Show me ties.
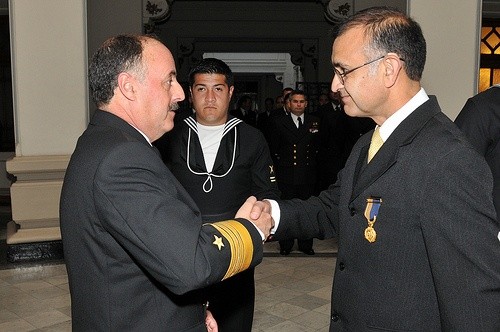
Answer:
[336,105,339,112]
[367,126,382,164]
[297,116,303,129]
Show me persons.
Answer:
[228,87,348,255]
[249,6,500,332]
[161,58,283,332]
[58,33,276,332]
[454,83,500,216]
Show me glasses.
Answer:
[333,55,407,85]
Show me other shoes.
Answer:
[280,249,290,255]
[305,249,314,255]
